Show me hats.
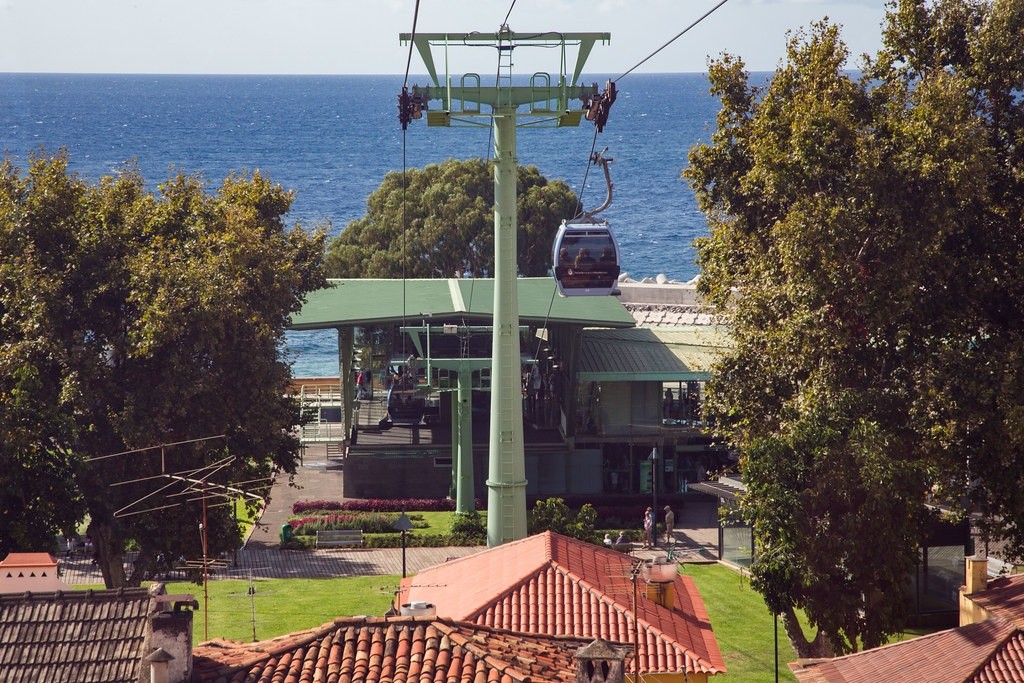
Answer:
[664,506,670,509]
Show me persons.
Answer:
[555,247,572,273]
[599,446,742,497]
[603,532,613,545]
[380,354,562,433]
[663,391,671,423]
[355,370,369,402]
[645,506,657,543]
[616,531,630,544]
[641,511,653,550]
[64,536,192,579]
[665,388,676,420]
[600,246,619,273]
[663,505,679,545]
[574,246,598,271]
[575,248,587,268]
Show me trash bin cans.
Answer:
[282,523,293,545]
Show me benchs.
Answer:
[316,529,363,551]
[986,556,1018,578]
[610,543,634,557]
